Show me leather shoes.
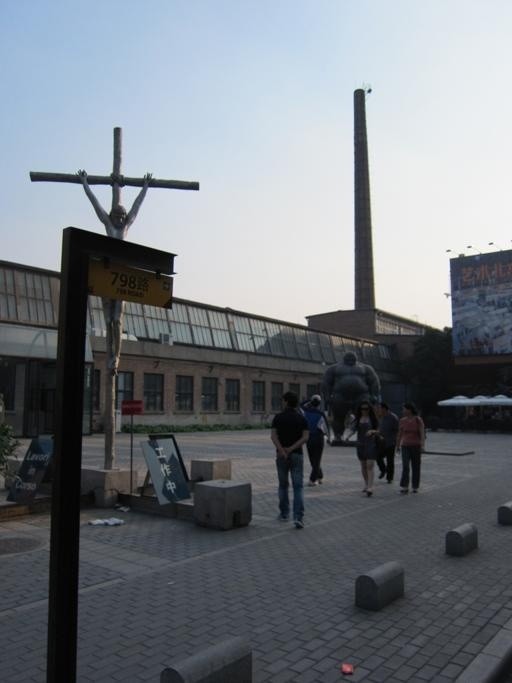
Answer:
[379,467,386,479]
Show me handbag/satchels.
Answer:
[374,432,386,455]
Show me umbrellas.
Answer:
[437,394,512,419]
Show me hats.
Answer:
[311,394,321,404]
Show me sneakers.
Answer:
[293,519,304,528]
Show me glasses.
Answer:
[360,406,368,411]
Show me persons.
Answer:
[394,399,426,494]
[319,350,384,441]
[299,394,330,486]
[344,398,377,497]
[74,166,159,375]
[374,401,399,485]
[270,390,311,529]
